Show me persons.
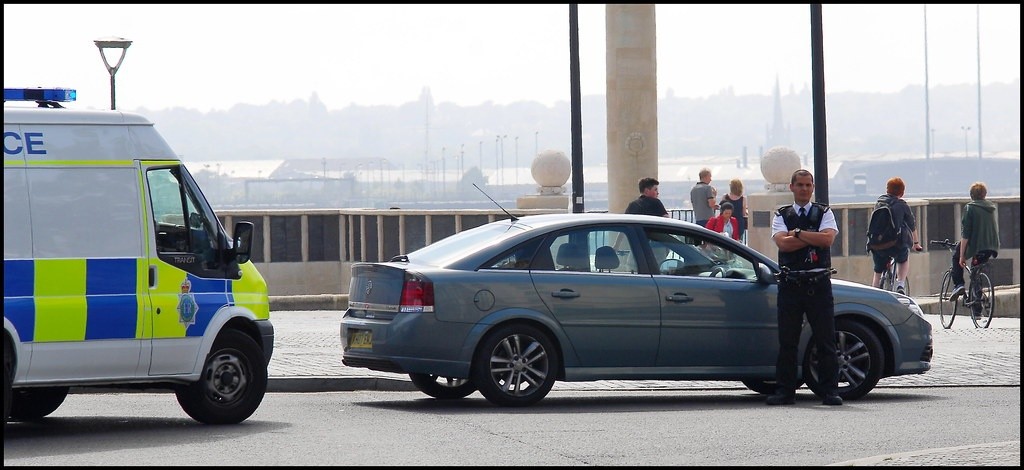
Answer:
[706,200,740,243]
[949,182,1000,320]
[690,168,720,228]
[719,177,747,239]
[767,169,845,406]
[866,178,922,295]
[611,176,671,265]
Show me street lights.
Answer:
[92,35,134,111]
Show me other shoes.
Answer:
[897,285,905,294]
[767,393,794,404]
[823,393,842,405]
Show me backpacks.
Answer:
[866,200,902,252]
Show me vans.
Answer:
[0,87,274,425]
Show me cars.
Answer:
[340,213,933,408]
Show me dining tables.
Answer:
[595,246,620,274]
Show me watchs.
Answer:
[795,228,801,238]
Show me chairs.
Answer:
[556,243,579,272]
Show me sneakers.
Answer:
[971,312,980,319]
[949,285,965,302]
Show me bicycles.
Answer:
[930,239,995,329]
[880,247,923,297]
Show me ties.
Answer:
[799,208,806,221]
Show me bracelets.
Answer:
[914,241,919,244]
